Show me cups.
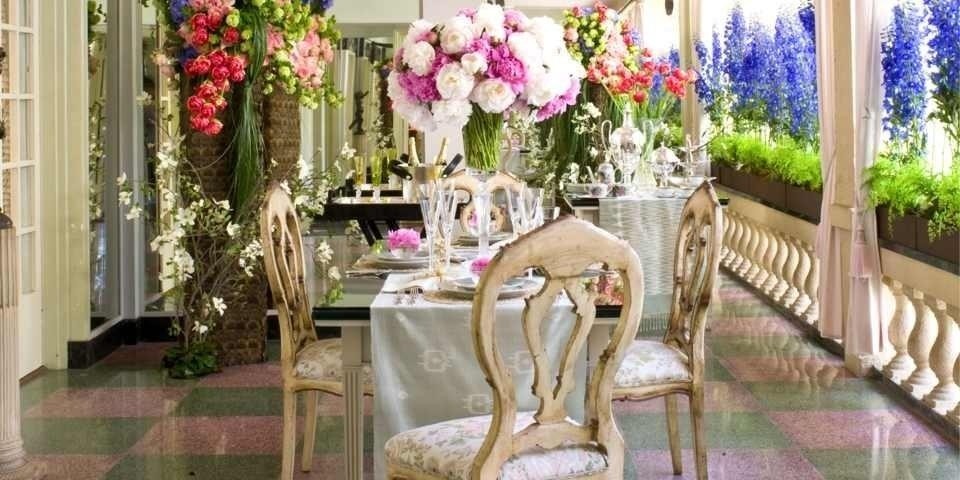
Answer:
[583,183,609,198]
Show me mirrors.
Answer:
[312,27,528,192]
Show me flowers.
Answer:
[383,3,583,170]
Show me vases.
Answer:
[915,216,960,264]
[164,346,222,378]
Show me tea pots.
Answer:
[588,158,623,197]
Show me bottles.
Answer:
[406,137,422,167]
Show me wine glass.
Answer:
[416,180,544,275]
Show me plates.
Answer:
[357,231,542,298]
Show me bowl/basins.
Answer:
[655,175,707,198]
